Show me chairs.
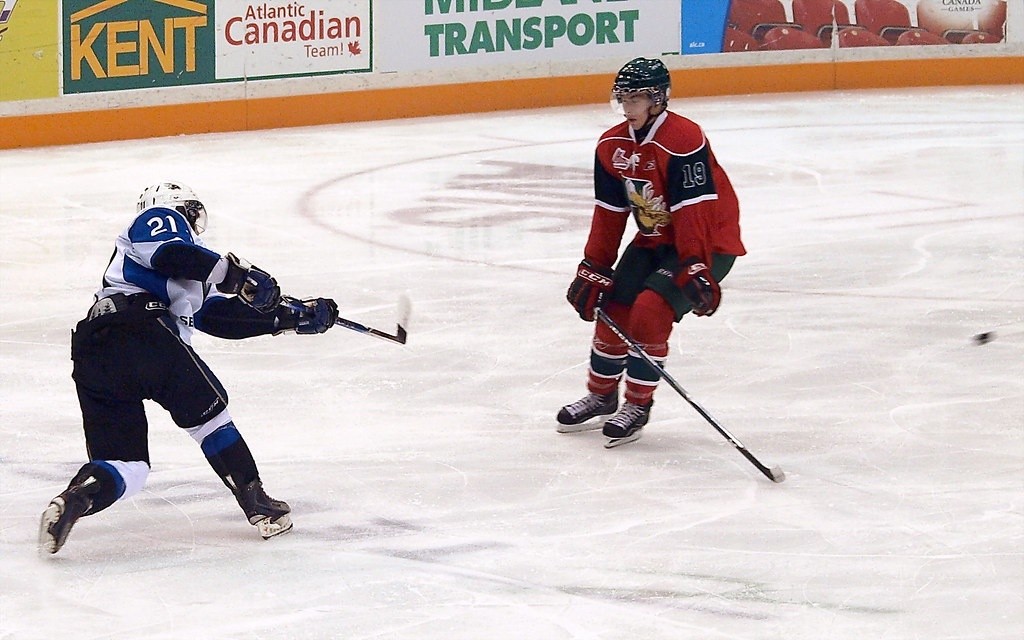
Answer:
[723,0,1005,57]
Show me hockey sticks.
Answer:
[594,306,786,484]
[279,288,412,348]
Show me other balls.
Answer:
[972,331,1000,346]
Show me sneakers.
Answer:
[556,390,619,433]
[39,485,88,554]
[602,400,654,449]
[233,478,293,539]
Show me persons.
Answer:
[556,57,745,450]
[36,180,338,556]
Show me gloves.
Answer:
[273,295,339,334]
[217,253,281,313]
[566,259,614,322]
[675,257,721,317]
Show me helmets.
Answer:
[135,180,203,213]
[611,58,671,106]
